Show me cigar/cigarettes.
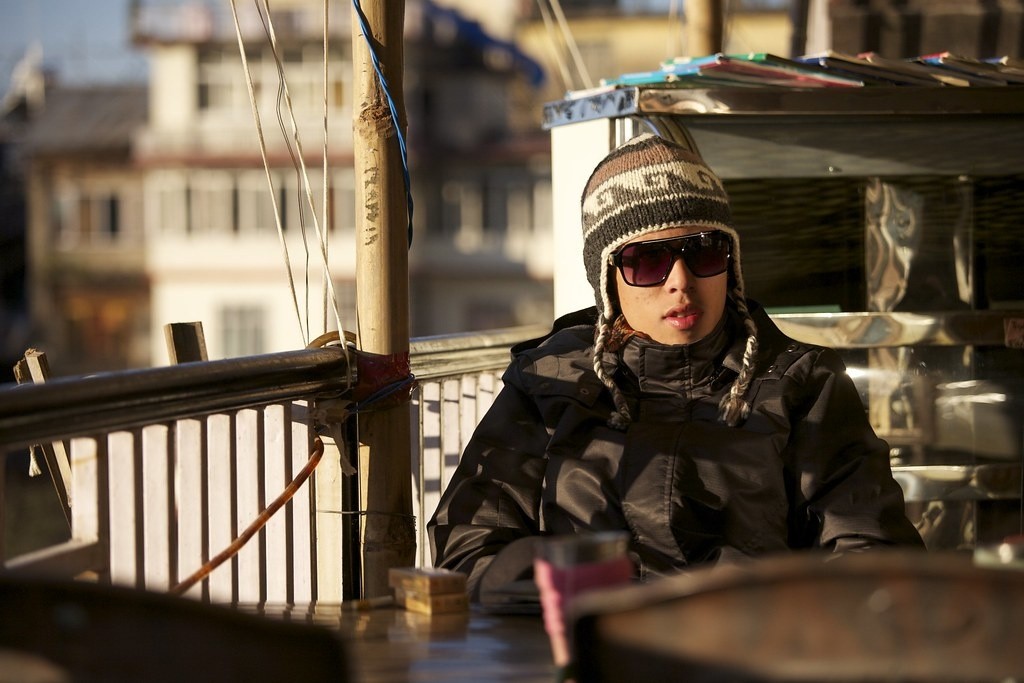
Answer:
[350,595,393,608]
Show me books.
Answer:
[565,50,1024,100]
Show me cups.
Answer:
[531,532,635,667]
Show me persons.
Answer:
[424,129,929,599]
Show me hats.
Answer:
[580,132,759,432]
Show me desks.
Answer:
[235,604,558,683]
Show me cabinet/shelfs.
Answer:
[542,86,1024,548]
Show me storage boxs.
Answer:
[388,566,468,614]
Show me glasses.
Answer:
[607,230,732,286]
